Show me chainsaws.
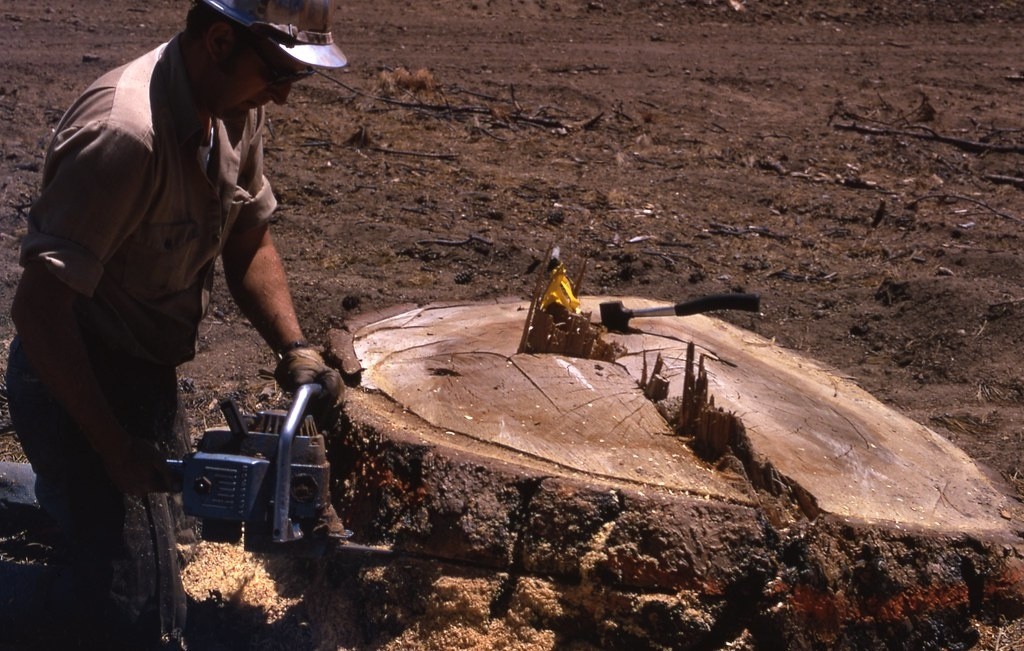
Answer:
[148,381,430,561]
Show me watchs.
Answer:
[272,339,310,364]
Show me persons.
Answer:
[7,0,349,651]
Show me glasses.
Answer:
[228,20,317,87]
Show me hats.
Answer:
[203,1,348,69]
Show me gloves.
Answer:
[274,348,347,413]
